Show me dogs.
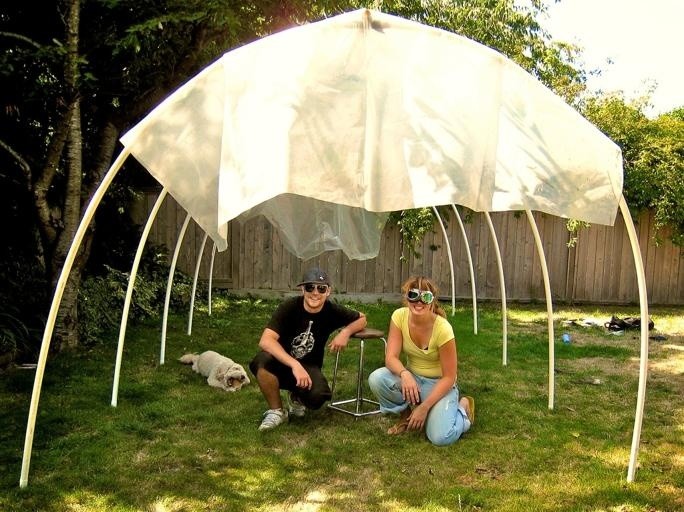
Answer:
[177,350,251,392]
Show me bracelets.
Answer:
[398,368,406,378]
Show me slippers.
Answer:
[387,419,410,435]
[462,395,476,427]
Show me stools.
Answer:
[325,328,389,419]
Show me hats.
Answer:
[296,267,330,287]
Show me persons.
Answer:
[366,275,474,448]
[246,267,366,432]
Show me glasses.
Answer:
[304,285,328,293]
[407,289,434,304]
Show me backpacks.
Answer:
[602,314,655,333]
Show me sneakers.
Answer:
[256,408,289,432]
[287,391,306,418]
